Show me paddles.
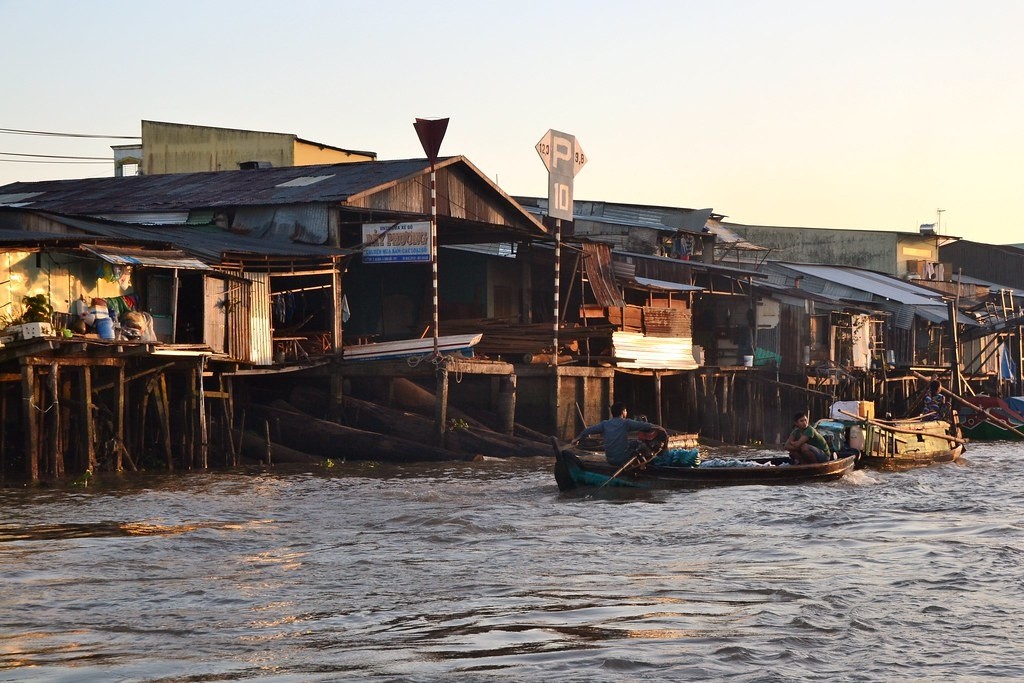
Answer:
[585,453,639,500]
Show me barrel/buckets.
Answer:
[744,355,753,367]
[96,318,115,339]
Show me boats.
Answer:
[816,409,968,473]
[553,444,861,497]
[956,395,1024,440]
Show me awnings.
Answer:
[342,202,555,250]
[88,249,214,272]
[916,305,983,329]
[222,247,368,260]
[752,280,885,307]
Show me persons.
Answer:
[571,403,669,467]
[927,381,946,419]
[784,413,831,464]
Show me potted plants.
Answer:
[20,294,54,340]
